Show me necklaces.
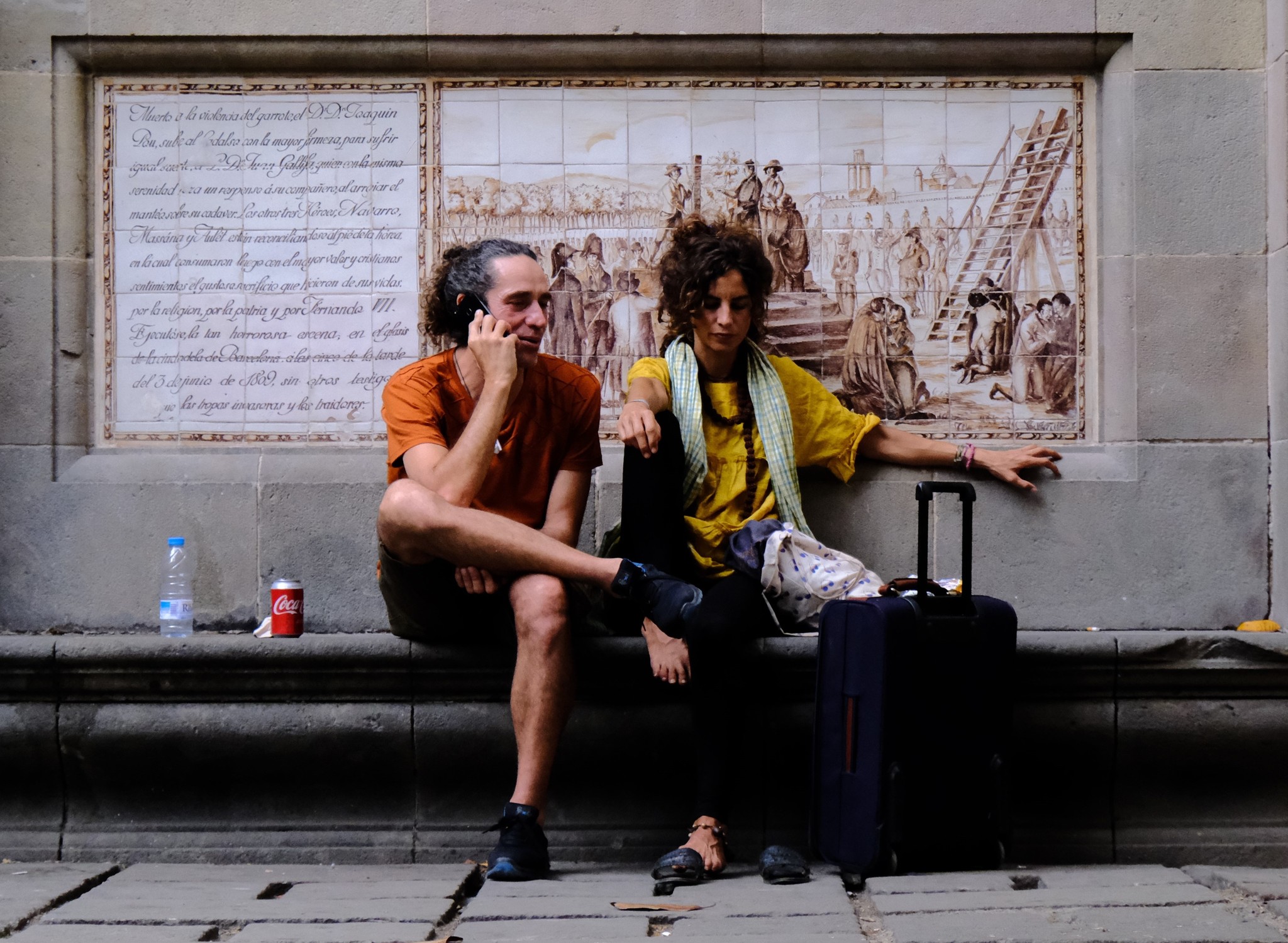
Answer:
[450,352,502,454]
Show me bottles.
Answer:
[159,537,194,638]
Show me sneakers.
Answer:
[482,801,551,882]
[609,557,703,631]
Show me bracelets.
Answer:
[954,441,974,470]
[627,398,653,410]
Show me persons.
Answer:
[615,216,1063,885]
[377,239,704,884]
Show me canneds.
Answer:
[271,578,304,638]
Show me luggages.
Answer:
[817,480,1024,890]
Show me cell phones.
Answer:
[453,293,511,338]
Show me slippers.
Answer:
[758,845,811,885]
[652,847,705,885]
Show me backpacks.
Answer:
[761,522,886,637]
